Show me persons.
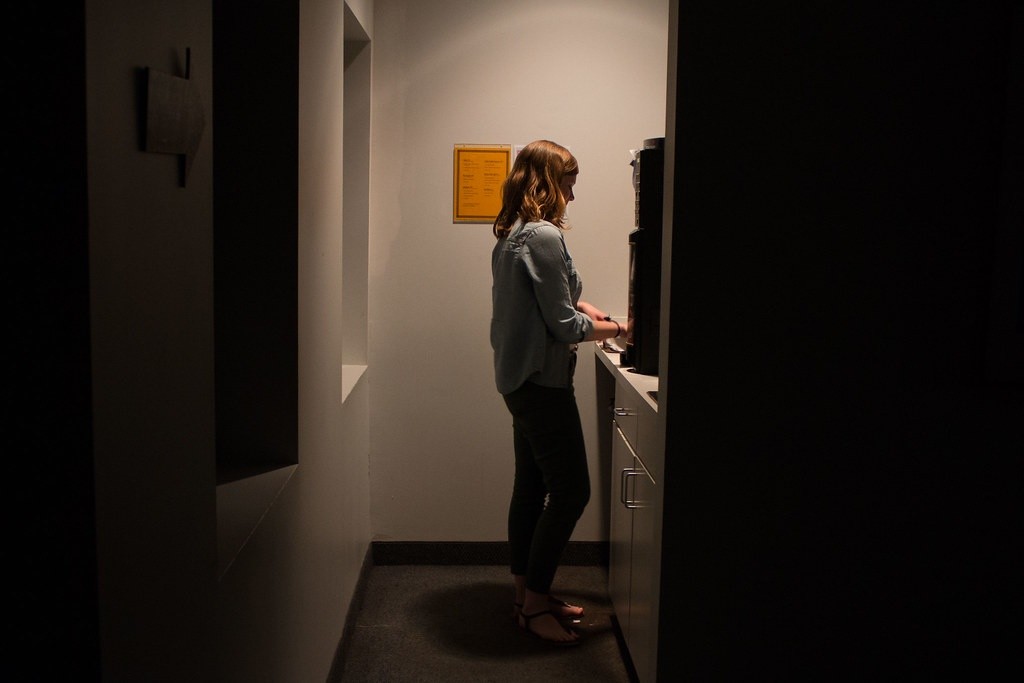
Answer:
[490,140,629,647]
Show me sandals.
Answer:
[518,609,581,646]
[511,596,584,623]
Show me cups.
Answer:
[620,351,626,364]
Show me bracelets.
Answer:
[603,316,621,338]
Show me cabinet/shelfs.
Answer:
[607,378,664,683]
